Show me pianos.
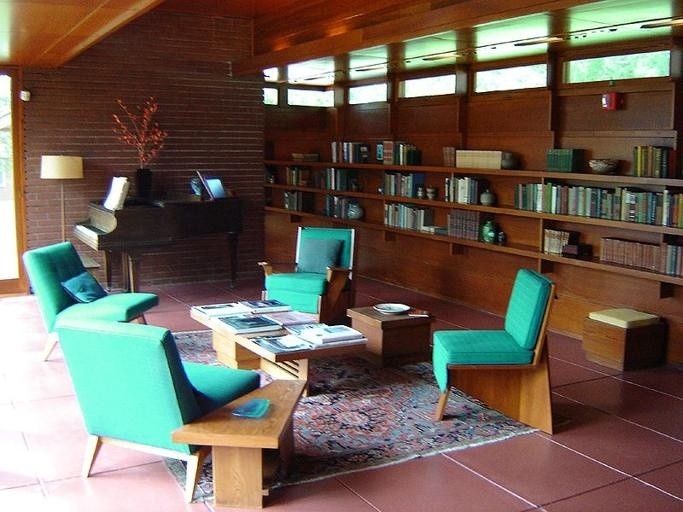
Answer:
[71,188,245,293]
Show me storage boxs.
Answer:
[545,149,583,173]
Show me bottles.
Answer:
[417,186,437,199]
[483,220,507,244]
[480,190,495,207]
[501,150,519,168]
[347,203,363,219]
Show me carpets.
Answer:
[162,331,571,501]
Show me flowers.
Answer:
[112,95,165,168]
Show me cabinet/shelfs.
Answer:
[265,160,683,365]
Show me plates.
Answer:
[373,303,411,316]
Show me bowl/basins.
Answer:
[588,158,621,176]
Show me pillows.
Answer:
[61,271,107,303]
[297,237,343,274]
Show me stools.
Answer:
[80,254,101,283]
[582,308,661,372]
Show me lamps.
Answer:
[40,154,84,243]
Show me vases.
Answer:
[135,169,152,199]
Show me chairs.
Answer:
[21,242,158,361]
[57,320,260,504]
[257,226,359,323]
[431,267,557,436]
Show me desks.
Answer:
[172,379,308,508]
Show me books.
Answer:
[589,308,660,329]
[514,183,543,211]
[547,148,583,172]
[286,167,312,186]
[383,171,423,197]
[544,228,579,254]
[102,175,130,212]
[599,237,682,276]
[453,177,488,203]
[284,191,312,211]
[633,146,682,178]
[544,183,682,229]
[408,309,433,319]
[326,168,357,191]
[384,203,446,232]
[450,210,490,240]
[326,196,355,218]
[192,299,367,356]
[456,150,505,170]
[338,142,422,166]
